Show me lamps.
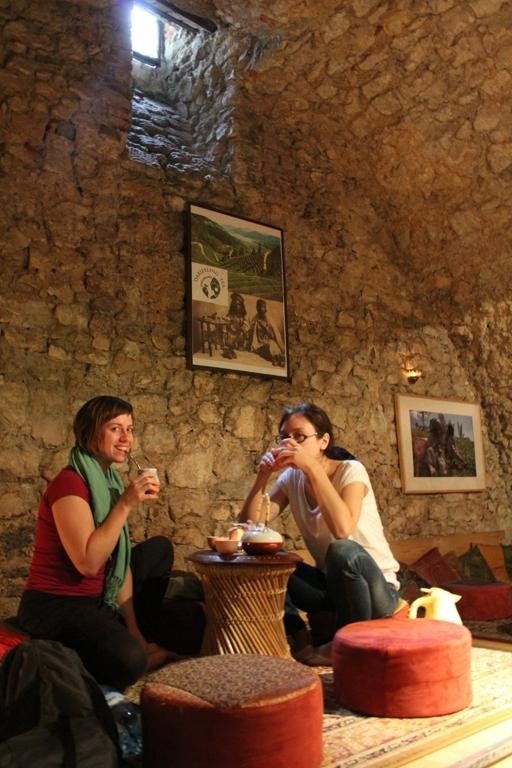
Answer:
[406,370,422,384]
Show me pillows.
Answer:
[500,544,512,579]
[457,546,496,585]
[408,547,462,586]
[442,550,465,580]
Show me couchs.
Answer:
[388,530,512,620]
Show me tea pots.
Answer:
[241,494,284,557]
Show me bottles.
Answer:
[105,691,143,760]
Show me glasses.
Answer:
[275,434,317,445]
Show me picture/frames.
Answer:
[395,391,486,494]
[184,200,290,379]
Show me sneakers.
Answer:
[290,641,333,666]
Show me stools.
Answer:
[331,618,472,718]
[139,653,325,768]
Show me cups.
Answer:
[216,539,240,556]
[208,536,228,551]
[139,468,157,494]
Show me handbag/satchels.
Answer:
[0,639,123,767]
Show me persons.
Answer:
[12,393,177,682]
[421,417,448,476]
[249,299,285,360]
[238,401,402,666]
[444,423,469,471]
[224,293,251,351]
